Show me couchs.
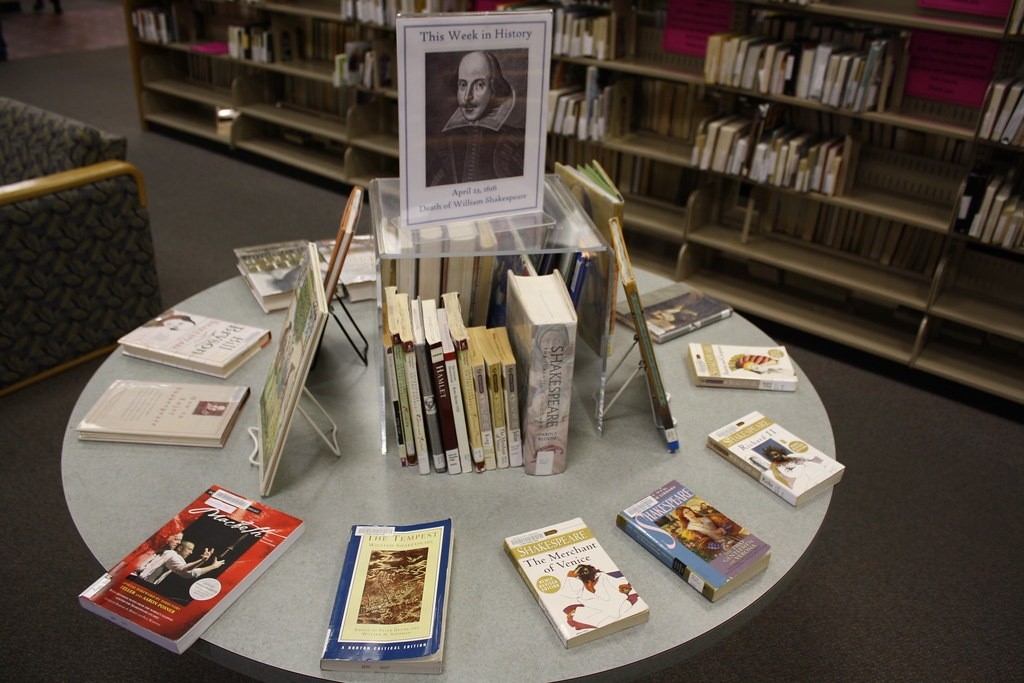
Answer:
[0,96,164,394]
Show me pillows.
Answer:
[0,97,100,186]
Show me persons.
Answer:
[127,532,225,596]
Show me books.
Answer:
[707,411,845,506]
[502,517,649,649]
[319,517,455,675]
[130,0,469,136]
[233,161,731,494]
[689,343,798,392]
[616,479,771,603]
[78,485,305,654]
[548,0,1024,283]
[76,379,251,449]
[117,309,271,379]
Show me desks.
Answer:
[61,266,836,683]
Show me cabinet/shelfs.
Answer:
[516,0,1024,402]
[122,0,466,199]
